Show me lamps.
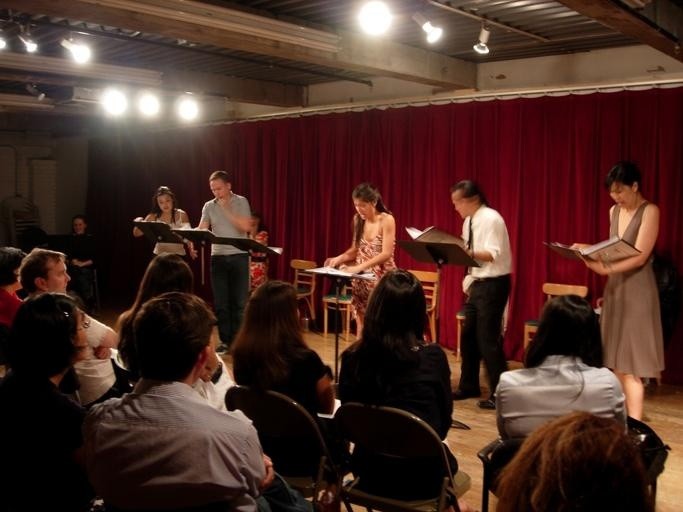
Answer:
[0,13,96,63]
[408,11,442,45]
[470,16,498,56]
[22,79,47,102]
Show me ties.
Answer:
[464,220,471,277]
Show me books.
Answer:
[403,225,463,252]
[130,219,178,243]
[168,226,216,244]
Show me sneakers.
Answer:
[215,343,229,355]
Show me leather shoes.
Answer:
[452,387,481,400]
[478,391,496,409]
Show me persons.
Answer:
[245,212,268,293]
[0,234,660,512]
[65,216,97,319]
[572,160,666,422]
[131,185,197,263]
[323,184,396,340]
[450,180,513,409]
[199,170,253,357]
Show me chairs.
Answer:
[521,281,592,353]
[407,267,439,344]
[321,258,360,339]
[289,258,318,330]
[218,378,471,509]
[475,411,675,510]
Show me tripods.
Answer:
[435,267,471,430]
